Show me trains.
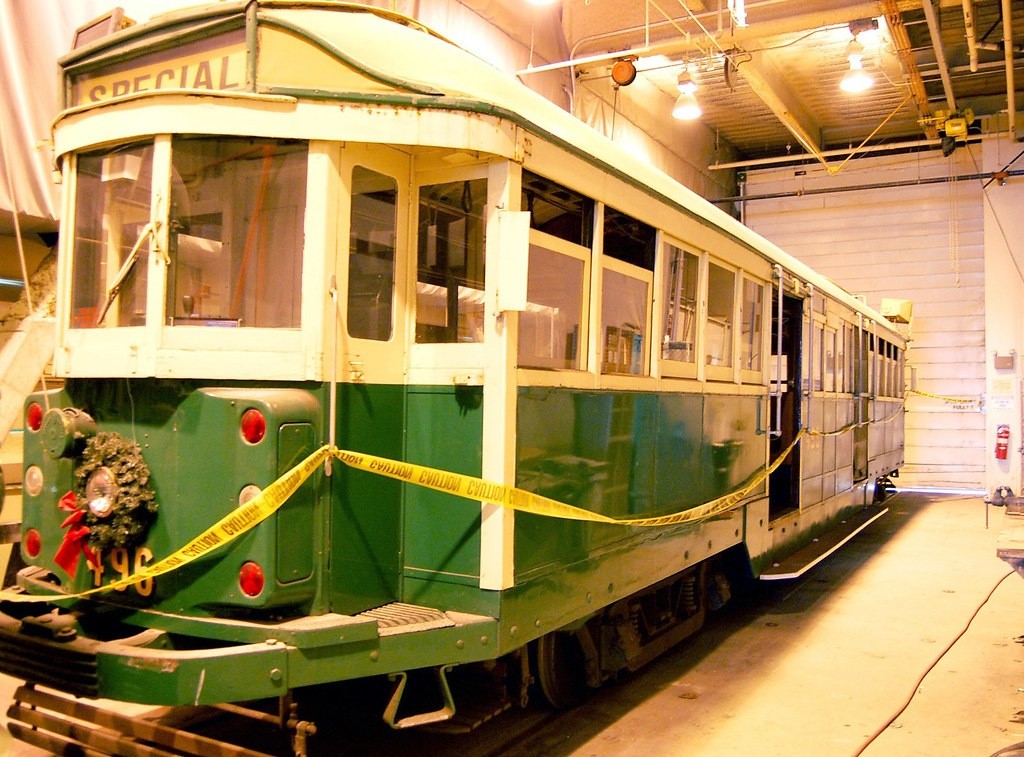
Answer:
[0,1,915,757]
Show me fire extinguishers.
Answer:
[995,424,1010,460]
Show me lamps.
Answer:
[837,41,872,94]
[671,71,703,120]
